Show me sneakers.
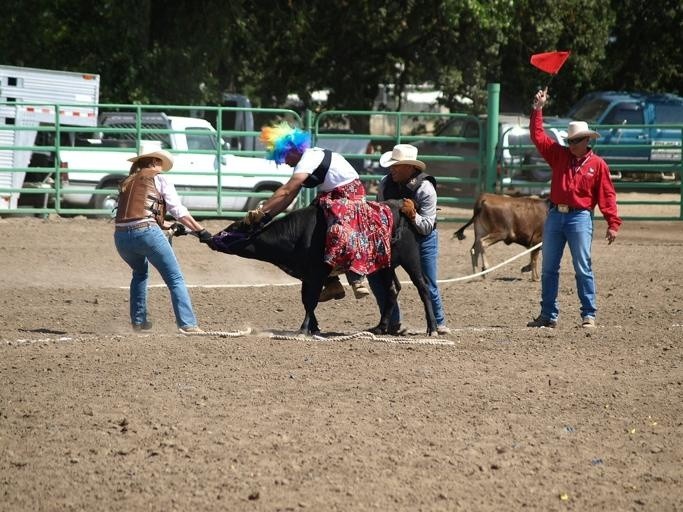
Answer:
[526,315,557,327]
[352,280,370,299]
[179,324,206,334]
[132,320,153,332]
[363,322,407,335]
[317,281,345,302]
[425,324,452,335]
[582,316,596,329]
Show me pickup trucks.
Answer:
[24,112,301,222]
[162,91,373,188]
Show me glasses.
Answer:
[562,136,587,145]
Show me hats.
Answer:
[379,143,427,171]
[126,151,173,172]
[561,120,601,141]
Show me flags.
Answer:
[530,50,572,75]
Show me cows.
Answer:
[453,192,550,282]
[205,203,440,338]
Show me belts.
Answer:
[549,200,585,214]
[115,220,157,232]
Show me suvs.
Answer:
[522,91,682,181]
[407,113,574,200]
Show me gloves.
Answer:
[195,227,212,243]
[168,222,189,237]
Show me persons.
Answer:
[244,121,395,302]
[368,143,451,338]
[114,152,212,335]
[526,86,622,329]
